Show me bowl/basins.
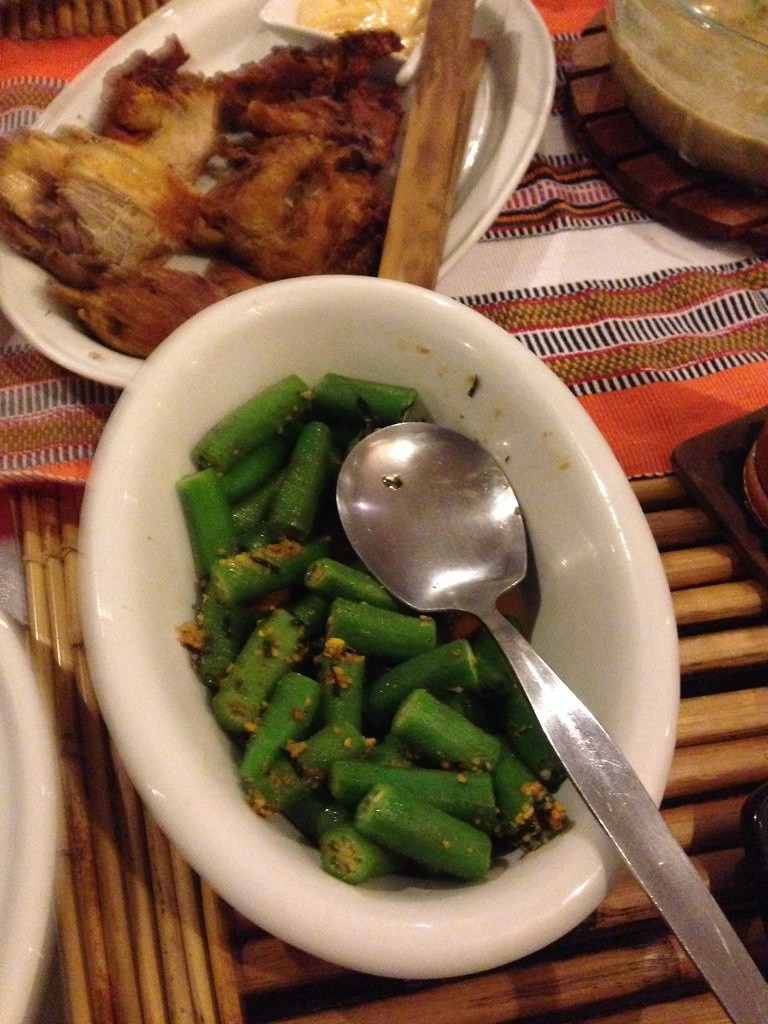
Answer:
[74,275,680,983]
[602,0,767,188]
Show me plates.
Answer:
[0,610,69,1024]
[0,0,555,386]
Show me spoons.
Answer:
[334,420,768,1024]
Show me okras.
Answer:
[177,371,567,886]
